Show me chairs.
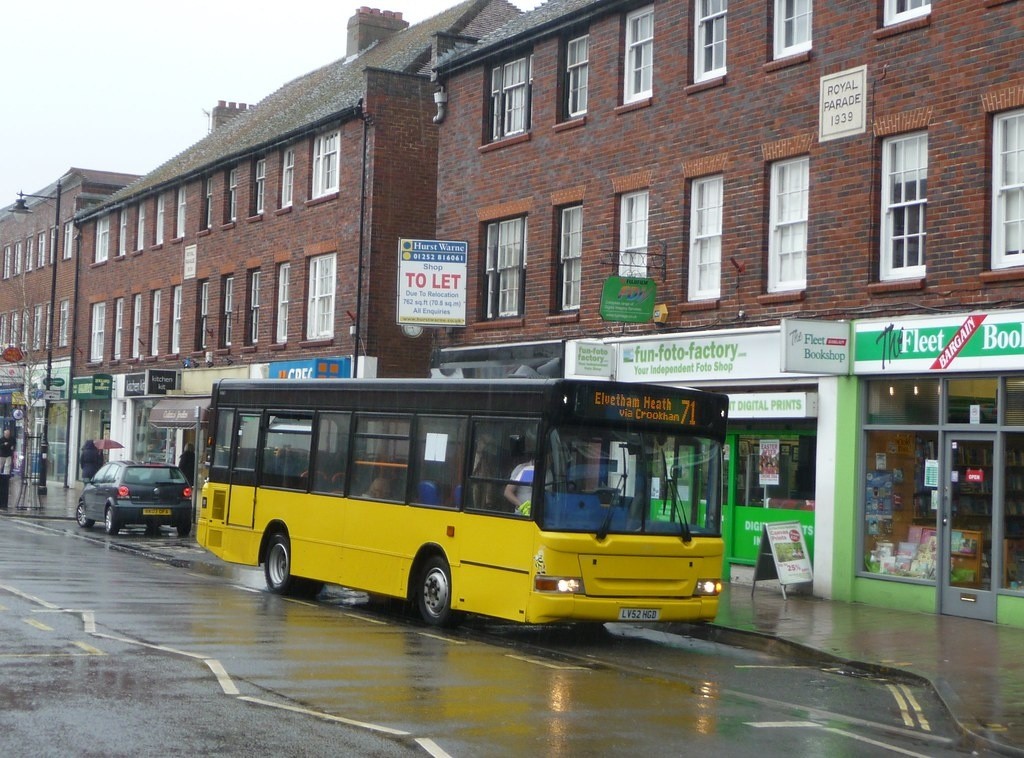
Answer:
[331,472,346,483]
[417,480,440,505]
[454,485,463,509]
[301,469,328,480]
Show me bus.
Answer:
[197,379,729,626]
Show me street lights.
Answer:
[7,181,62,495]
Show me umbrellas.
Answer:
[93,439,125,449]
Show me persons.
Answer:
[0,428,16,474]
[505,448,554,516]
[178,442,195,486]
[361,477,392,500]
[471,422,504,510]
[80,439,107,488]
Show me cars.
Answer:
[76,460,192,536]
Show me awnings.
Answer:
[148,398,211,430]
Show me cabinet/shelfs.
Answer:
[915,433,1024,592]
